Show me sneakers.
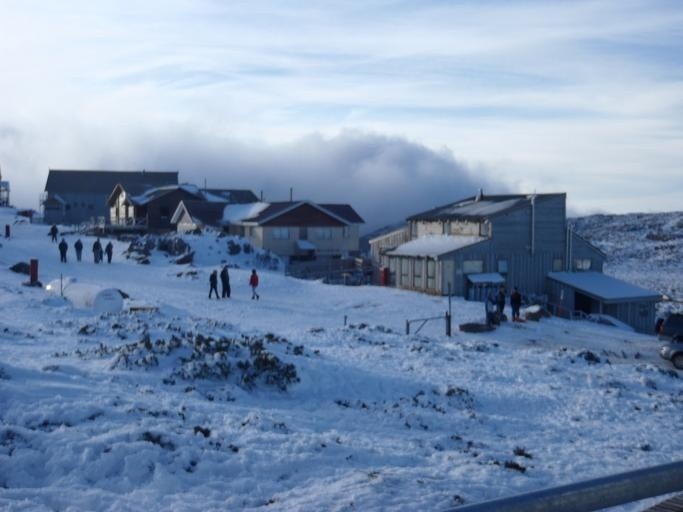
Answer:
[250,295,259,300]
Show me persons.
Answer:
[51,224,58,242]
[495,283,507,315]
[93,241,113,264]
[74,238,83,262]
[249,268,260,300]
[485,287,496,323]
[58,238,68,263]
[209,270,219,298]
[511,286,521,321]
[220,265,230,297]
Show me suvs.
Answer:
[654,313,683,342]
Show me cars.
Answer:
[658,334,683,370]
